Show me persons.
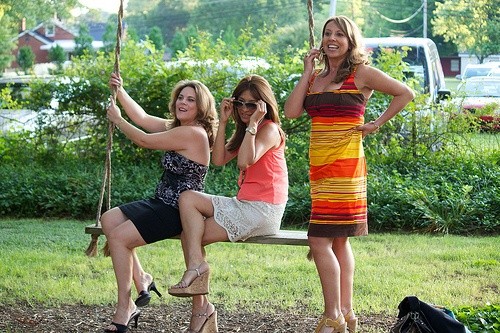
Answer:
[101,71,219,333]
[168,75,289,332]
[284,16,416,333]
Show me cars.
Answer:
[0,74,108,151]
[449,77,500,133]
[455,62,500,81]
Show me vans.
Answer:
[360,37,452,153]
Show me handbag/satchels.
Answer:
[389,296,472,333]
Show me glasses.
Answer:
[232,99,258,111]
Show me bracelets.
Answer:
[369,121,379,130]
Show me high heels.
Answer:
[133,281,163,307]
[103,301,142,333]
[168,260,210,297]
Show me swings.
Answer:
[85,0,315,263]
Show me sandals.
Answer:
[184,302,218,333]
[312,310,358,332]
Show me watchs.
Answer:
[245,126,257,135]
